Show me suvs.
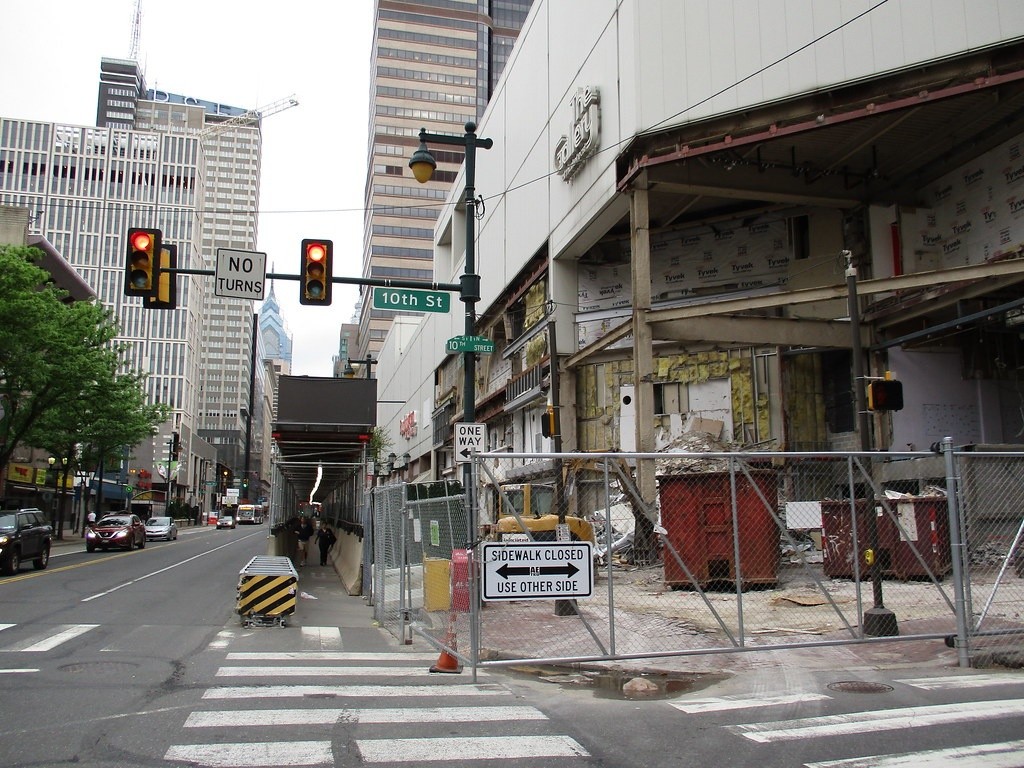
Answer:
[0,508,55,576]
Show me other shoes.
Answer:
[320,563,327,566]
[300,561,306,566]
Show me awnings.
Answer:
[89,479,133,500]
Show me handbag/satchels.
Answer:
[328,529,337,545]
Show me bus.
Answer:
[236,504,264,525]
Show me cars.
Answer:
[145,517,179,542]
[216,516,236,530]
[84,513,147,551]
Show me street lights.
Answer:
[47,456,68,540]
[114,473,131,513]
[409,120,494,612]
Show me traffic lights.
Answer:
[300,238,333,306]
[125,228,163,297]
[243,479,247,487]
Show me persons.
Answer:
[88,511,96,525]
[315,521,334,566]
[294,516,314,567]
